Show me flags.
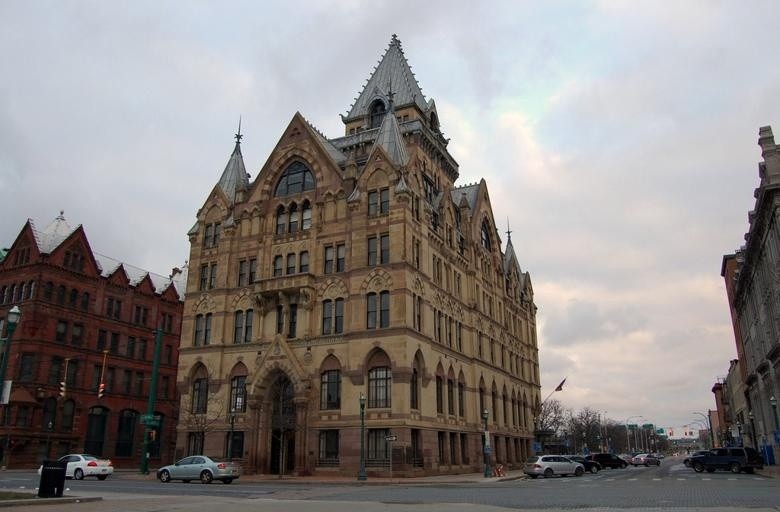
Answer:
[555,379,566,391]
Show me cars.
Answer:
[567,456,601,474]
[632,453,660,467]
[37,454,114,480]
[683,450,716,472]
[660,455,664,459]
[586,453,631,469]
[522,455,585,478]
[157,455,241,484]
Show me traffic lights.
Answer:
[97,383,106,401]
[57,377,66,401]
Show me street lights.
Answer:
[729,427,732,448]
[359,393,366,477]
[603,411,611,447]
[736,421,743,447]
[481,408,489,471]
[0,305,22,400]
[748,412,758,451]
[683,412,709,439]
[626,416,649,452]
[770,396,780,434]
[228,407,235,462]
[44,420,53,459]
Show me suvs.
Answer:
[688,447,762,473]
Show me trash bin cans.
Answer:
[38,460,68,497]
[763,445,775,466]
